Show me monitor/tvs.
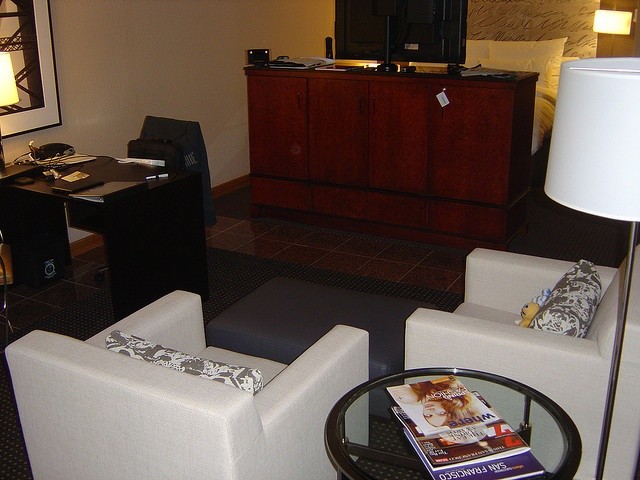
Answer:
[335,0,468,73]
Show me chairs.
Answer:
[95,115,201,281]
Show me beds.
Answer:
[464,37,579,157]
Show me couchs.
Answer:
[404,243,639,478]
[4,289,370,479]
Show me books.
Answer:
[389,391,532,472]
[402,427,545,479]
[51,170,104,193]
[385,376,499,436]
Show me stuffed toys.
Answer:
[518,302,540,328]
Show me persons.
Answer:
[394,376,471,427]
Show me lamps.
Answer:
[543,58,640,479]
[0,52,44,184]
[592,10,633,57]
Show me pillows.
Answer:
[527,258,602,339]
[105,329,263,397]
[478,56,535,71]
[488,37,568,76]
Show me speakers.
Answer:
[23,251,61,292]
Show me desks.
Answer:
[322,364,582,479]
[0,150,210,323]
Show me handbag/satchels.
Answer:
[127,138,184,169]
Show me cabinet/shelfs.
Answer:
[243,62,539,255]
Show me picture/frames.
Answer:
[0,0,62,140]
